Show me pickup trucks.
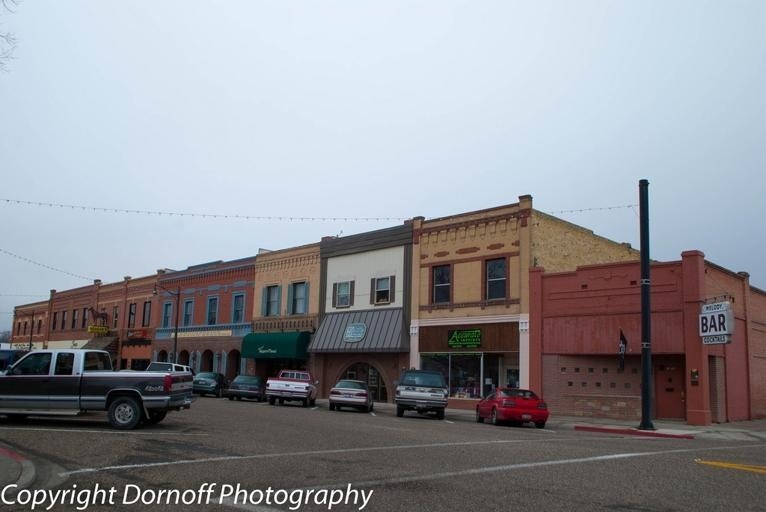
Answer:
[264,370,320,406]
[1,349,197,430]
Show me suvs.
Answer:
[393,368,450,419]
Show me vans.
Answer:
[145,361,196,382]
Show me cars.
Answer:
[476,388,549,428]
[193,372,225,397]
[227,375,266,401]
[329,379,376,413]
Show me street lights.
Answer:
[13,309,34,351]
[153,282,180,363]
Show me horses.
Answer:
[88,307,108,327]
